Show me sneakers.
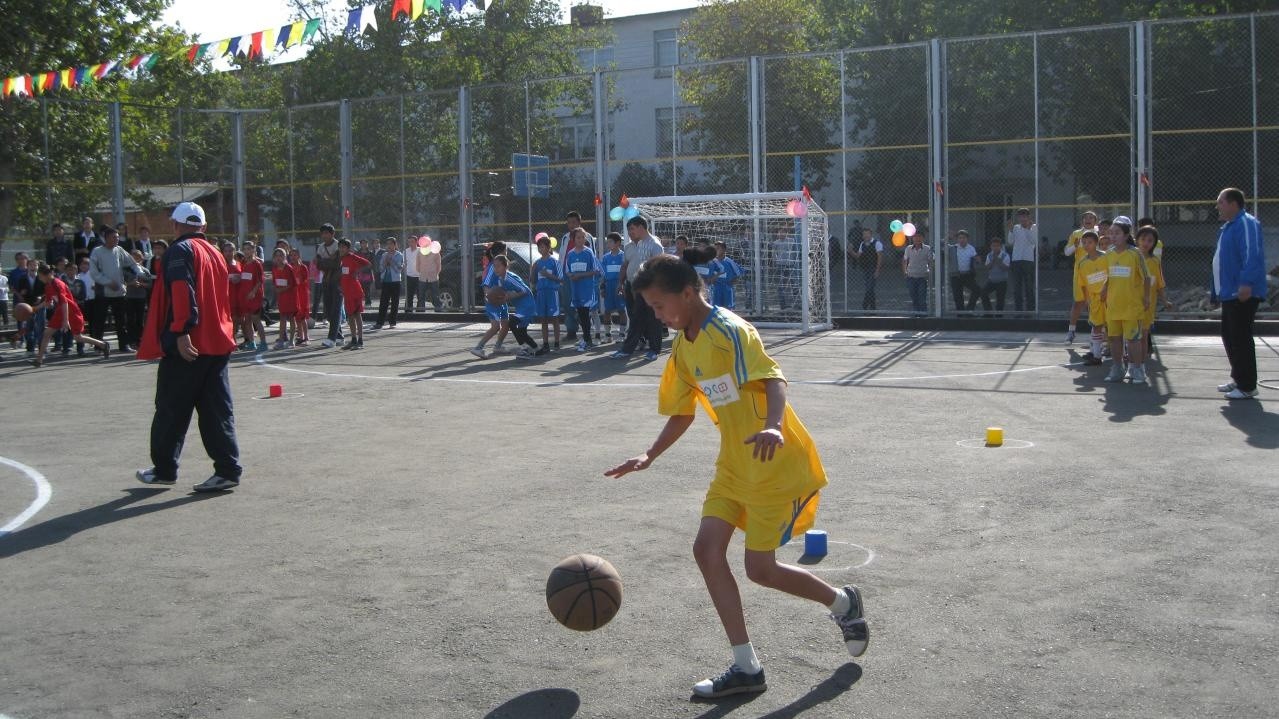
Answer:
[1065,333,1150,384]
[193,473,239,491]
[831,583,868,657]
[473,330,671,362]
[692,664,767,697]
[1217,382,1237,391]
[237,334,364,351]
[1224,389,1259,399]
[135,467,176,485]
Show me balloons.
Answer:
[419,236,441,255]
[535,233,548,242]
[548,237,557,248]
[787,199,808,218]
[890,220,916,246]
[610,206,640,220]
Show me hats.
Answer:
[169,201,206,225]
[1113,216,1132,226]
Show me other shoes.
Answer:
[372,324,383,329]
[390,321,396,328]
[9,337,136,359]
[26,359,41,366]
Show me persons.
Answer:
[980,237,1010,317]
[416,253,444,312]
[1007,207,1036,311]
[847,220,863,267]
[1209,187,1267,399]
[948,229,980,316]
[772,228,801,310]
[903,229,930,317]
[0,217,168,368]
[471,211,668,361]
[135,202,242,492]
[605,254,871,697]
[208,223,420,349]
[739,227,765,308]
[858,227,883,316]
[1064,211,1172,381]
[674,234,743,311]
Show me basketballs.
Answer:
[545,554,623,632]
[14,303,32,322]
[486,287,505,304]
